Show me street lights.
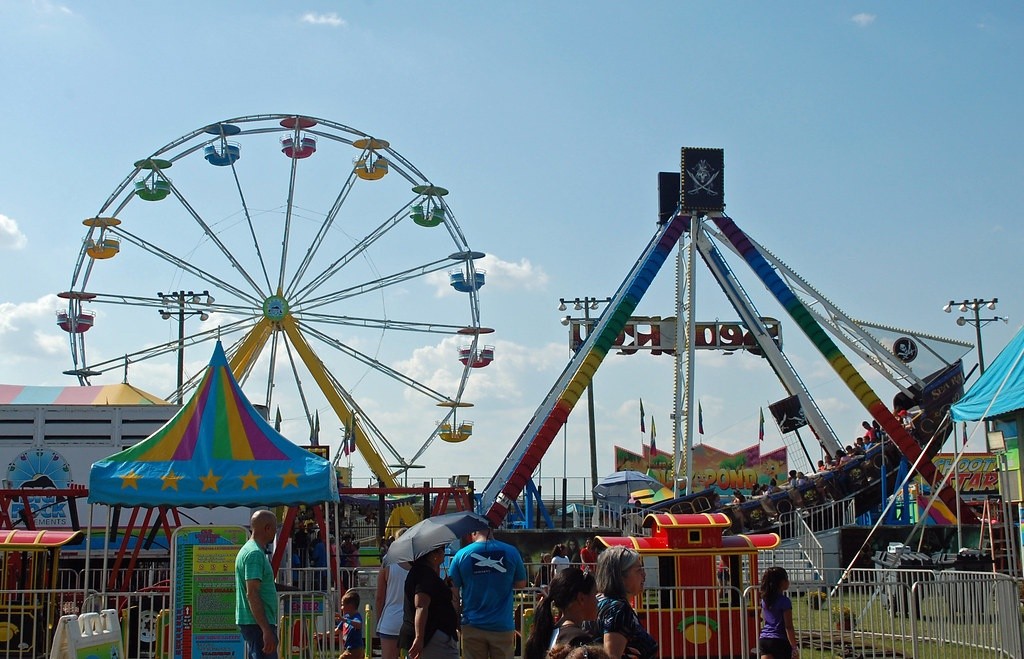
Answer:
[942,297,1010,453]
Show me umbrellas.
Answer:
[382,511,488,568]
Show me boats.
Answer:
[472,145,966,563]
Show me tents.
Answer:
[87,341,345,659]
[592,471,674,520]
[949,325,1024,550]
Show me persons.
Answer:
[549,643,612,659]
[716,555,730,597]
[447,515,527,659]
[757,566,800,659]
[314,592,365,659]
[712,404,915,536]
[290,526,395,590]
[525,567,600,659]
[375,527,415,659]
[582,546,659,659]
[396,544,461,659]
[532,535,599,588]
[235,511,280,659]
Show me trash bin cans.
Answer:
[932,551,997,618]
[872,550,938,618]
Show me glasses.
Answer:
[617,547,630,569]
